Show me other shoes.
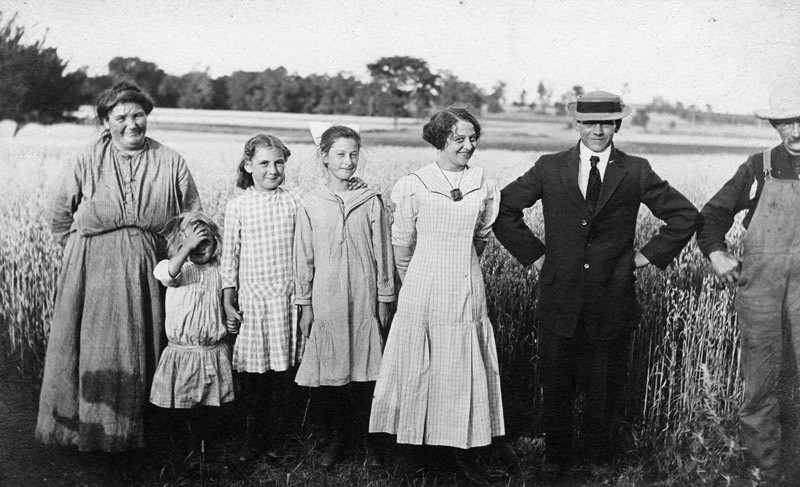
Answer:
[537,460,563,480]
[321,446,343,468]
[586,462,612,484]
[368,442,381,468]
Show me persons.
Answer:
[369,108,505,451]
[293,122,397,469]
[696,76,800,487]
[149,210,243,434]
[221,134,369,461]
[492,91,700,474]
[35,81,204,458]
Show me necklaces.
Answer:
[437,162,465,203]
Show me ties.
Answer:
[586,155,602,215]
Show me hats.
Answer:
[755,89,800,120]
[566,91,631,121]
